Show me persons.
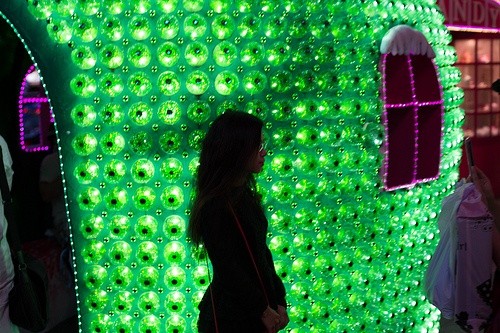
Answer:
[187,110,290,333]
[466,77,500,333]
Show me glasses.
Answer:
[257,141,267,151]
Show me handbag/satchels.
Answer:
[451,180,493,332]
[423,182,473,318]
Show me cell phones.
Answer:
[465,138,473,170]
